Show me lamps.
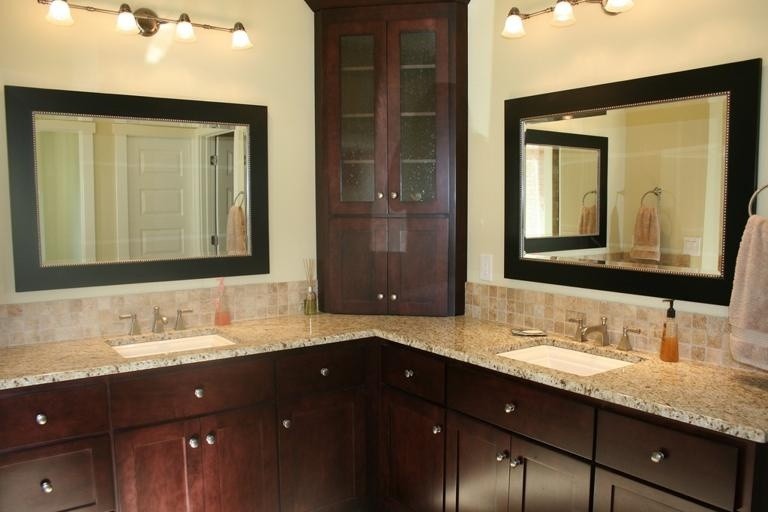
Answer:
[501,0,635,38]
[38,0,252,50]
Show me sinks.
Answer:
[108,331,240,359]
[494,338,646,377]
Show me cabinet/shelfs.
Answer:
[0,372,117,512]
[276,337,376,512]
[109,351,281,512]
[376,336,447,512]
[445,357,596,512]
[593,397,756,512]
[305,0,471,317]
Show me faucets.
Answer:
[152,306,169,333]
[578,316,611,345]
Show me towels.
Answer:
[630,203,661,263]
[728,215,768,371]
[579,204,597,233]
[226,206,247,257]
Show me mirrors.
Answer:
[504,57,762,305]
[4,85,270,293]
[524,129,608,254]
